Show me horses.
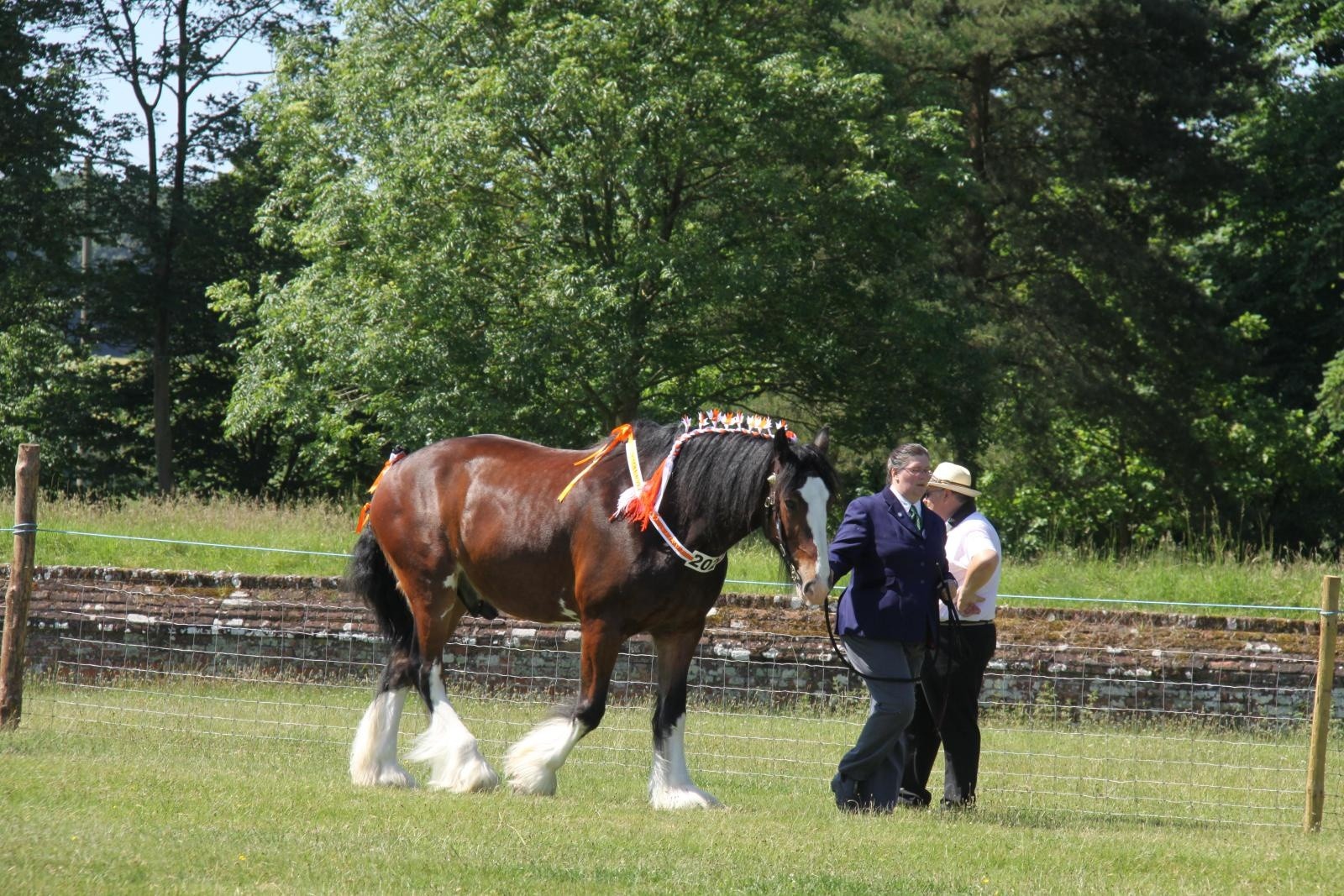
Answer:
[344,417,838,813]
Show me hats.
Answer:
[927,461,982,497]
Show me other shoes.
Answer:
[831,771,862,813]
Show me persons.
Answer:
[897,461,1002,814]
[827,443,959,816]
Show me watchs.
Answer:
[954,579,958,587]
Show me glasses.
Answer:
[899,465,932,477]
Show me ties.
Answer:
[909,505,921,530]
